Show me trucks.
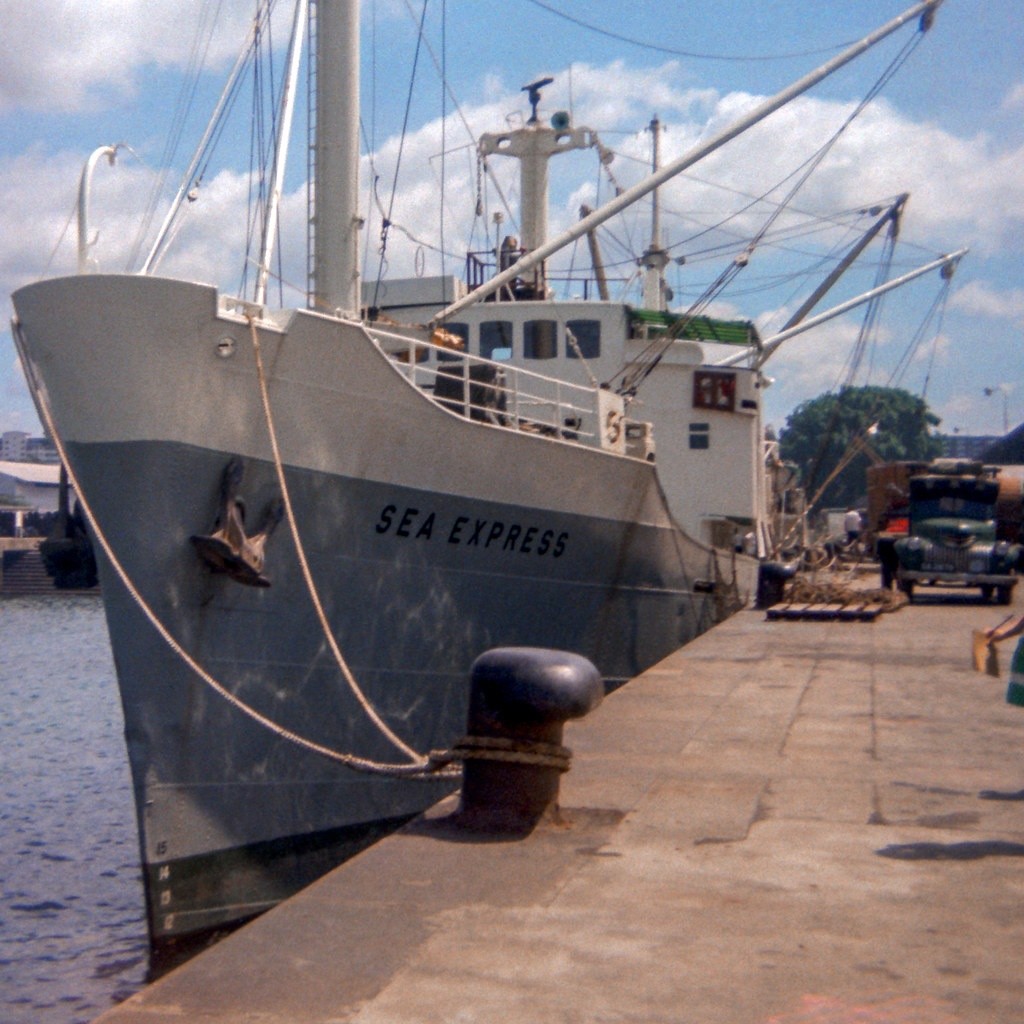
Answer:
[878,471,1019,606]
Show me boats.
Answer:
[6,3,983,972]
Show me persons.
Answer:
[845,504,862,552]
[731,527,745,554]
